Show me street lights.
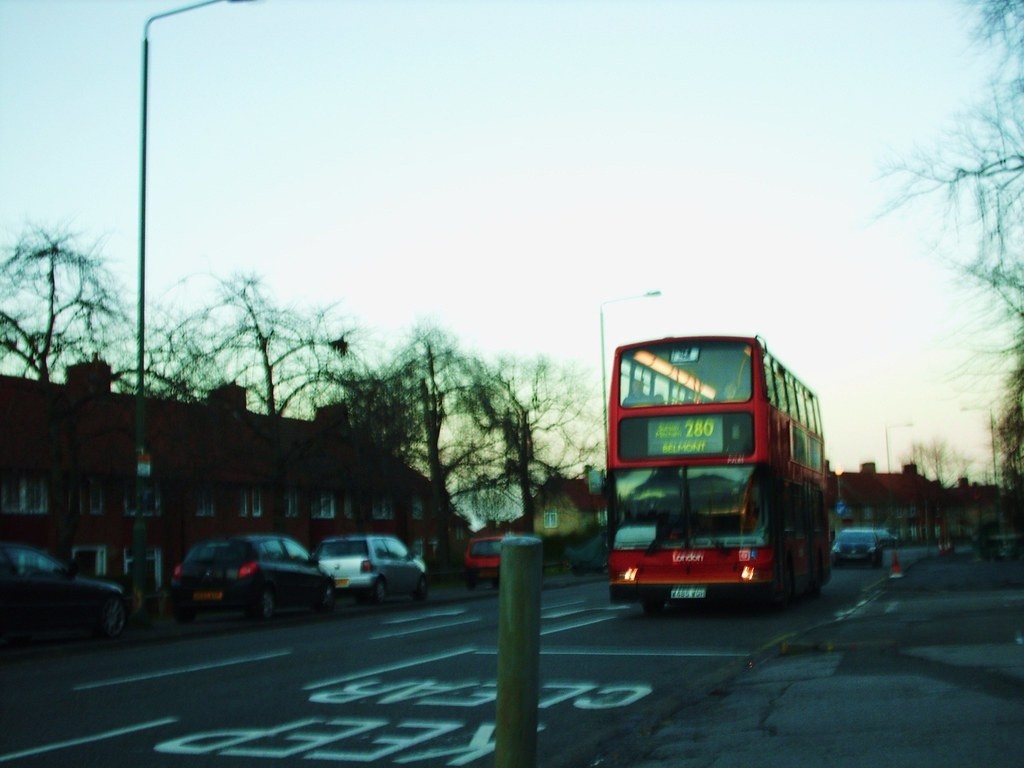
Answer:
[599,289,665,472]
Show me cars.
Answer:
[874,530,899,550]
[317,535,431,611]
[466,535,507,588]
[830,528,884,571]
[1,543,129,640]
[173,536,335,622]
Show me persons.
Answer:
[623,380,656,407]
[716,383,744,402]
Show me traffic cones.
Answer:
[889,548,904,578]
[939,537,954,557]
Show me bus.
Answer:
[605,334,835,619]
